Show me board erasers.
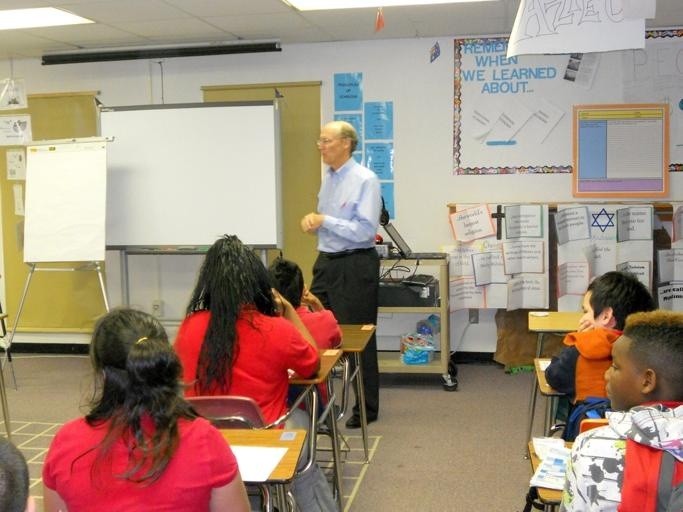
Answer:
[177,246,198,250]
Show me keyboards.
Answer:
[402,275,434,286]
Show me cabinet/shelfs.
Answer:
[377,253,458,390]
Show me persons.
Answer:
[0,434,37,512]
[42,306,255,512]
[543,270,658,441]
[172,234,342,512]
[558,309,683,512]
[299,120,381,429]
[266,254,343,420]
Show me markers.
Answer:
[196,247,210,251]
[141,247,176,250]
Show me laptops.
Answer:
[384,223,447,259]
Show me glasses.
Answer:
[314,137,337,147]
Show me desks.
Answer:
[525,312,585,512]
[177,323,378,512]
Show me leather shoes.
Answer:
[345,411,376,430]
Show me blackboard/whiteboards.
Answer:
[96,99,283,254]
[23,137,114,262]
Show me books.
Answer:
[529,446,572,492]
